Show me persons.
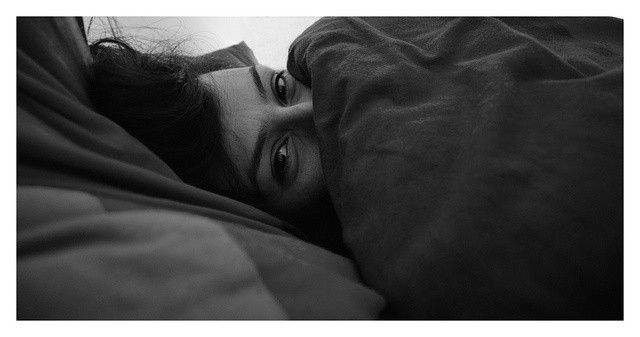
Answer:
[81,19,338,247]
